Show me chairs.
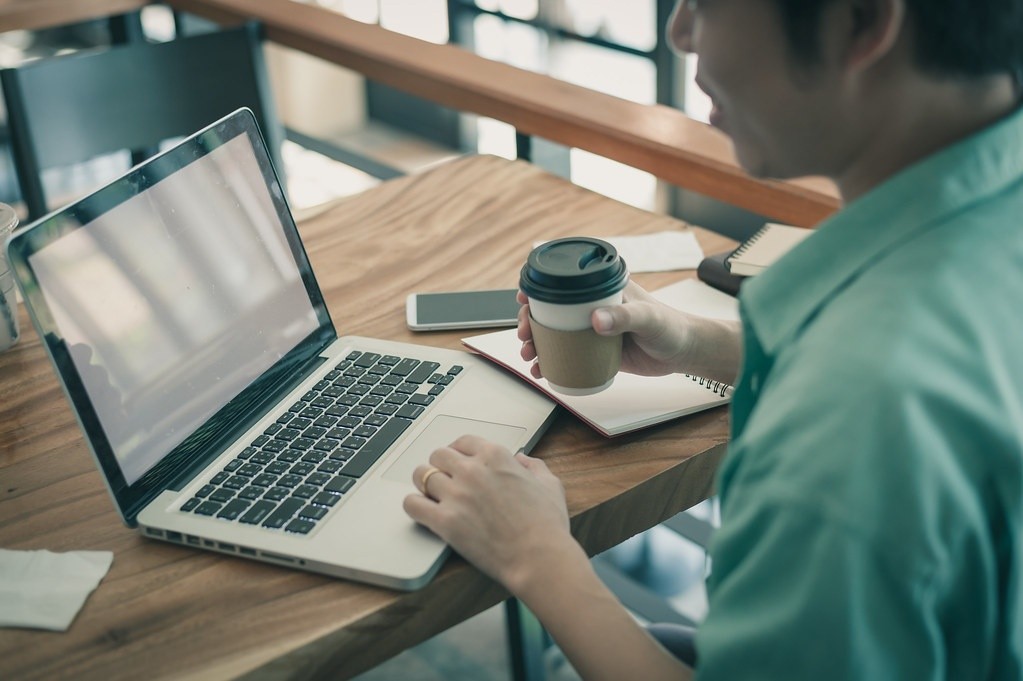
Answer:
[0,21,290,221]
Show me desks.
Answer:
[0,153,743,681]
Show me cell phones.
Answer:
[406,288,523,332]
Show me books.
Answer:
[462,219,816,440]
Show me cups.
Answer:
[521,236,631,397]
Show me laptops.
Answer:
[3,106,560,591]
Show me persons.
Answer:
[400,0,1023,681]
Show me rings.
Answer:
[421,468,440,496]
